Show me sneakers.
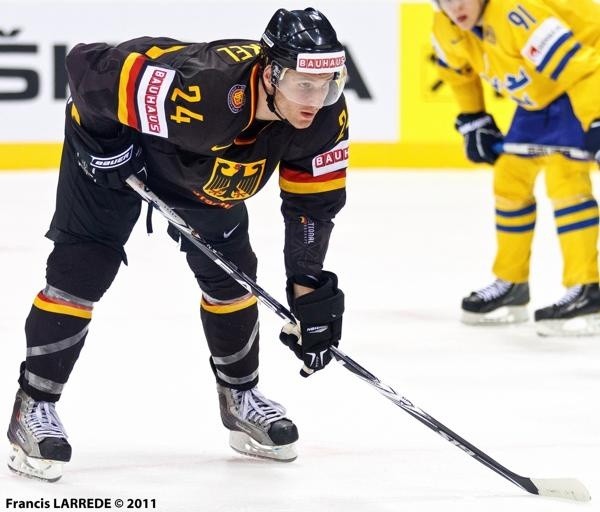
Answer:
[462,277,530,313]
[535,282,599,319]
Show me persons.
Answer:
[431,0,600,322]
[6,7,349,463]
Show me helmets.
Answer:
[258,7,348,107]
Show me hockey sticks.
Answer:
[127,173,592,503]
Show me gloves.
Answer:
[581,118,600,163]
[280,271,343,377]
[455,112,504,164]
[68,116,150,193]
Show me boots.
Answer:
[7,359,72,462]
[209,354,298,446]
